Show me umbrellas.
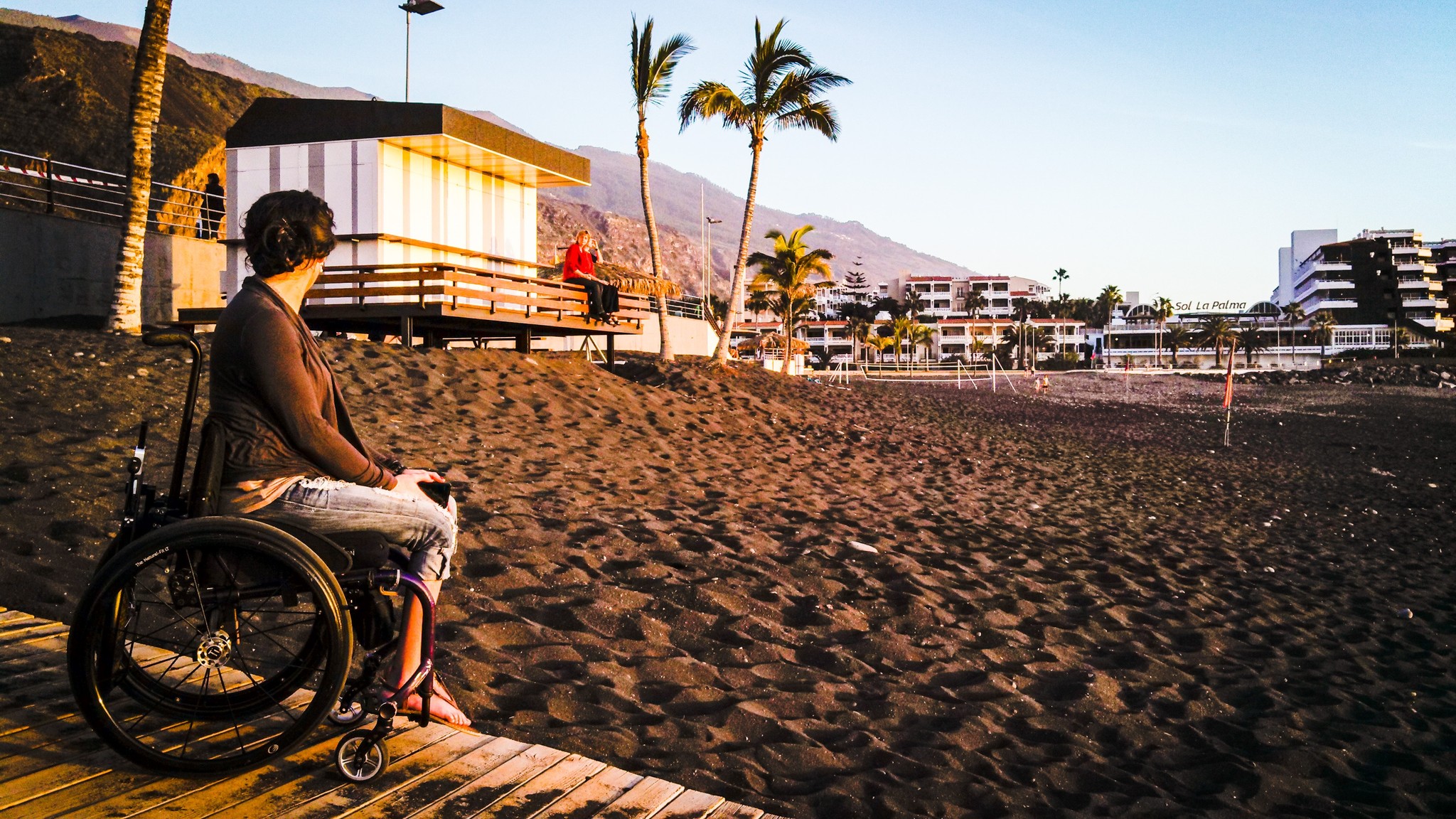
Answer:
[738,332,810,372]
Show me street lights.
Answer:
[399,0,446,102]
[706,216,723,316]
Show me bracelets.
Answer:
[594,248,598,251]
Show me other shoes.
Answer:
[588,312,603,321]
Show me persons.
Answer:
[563,229,622,326]
[1024,363,1049,393]
[211,190,476,734]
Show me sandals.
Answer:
[604,314,615,325]
[364,670,479,736]
[611,315,621,326]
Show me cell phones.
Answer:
[418,481,452,509]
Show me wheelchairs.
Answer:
[65,326,483,787]
